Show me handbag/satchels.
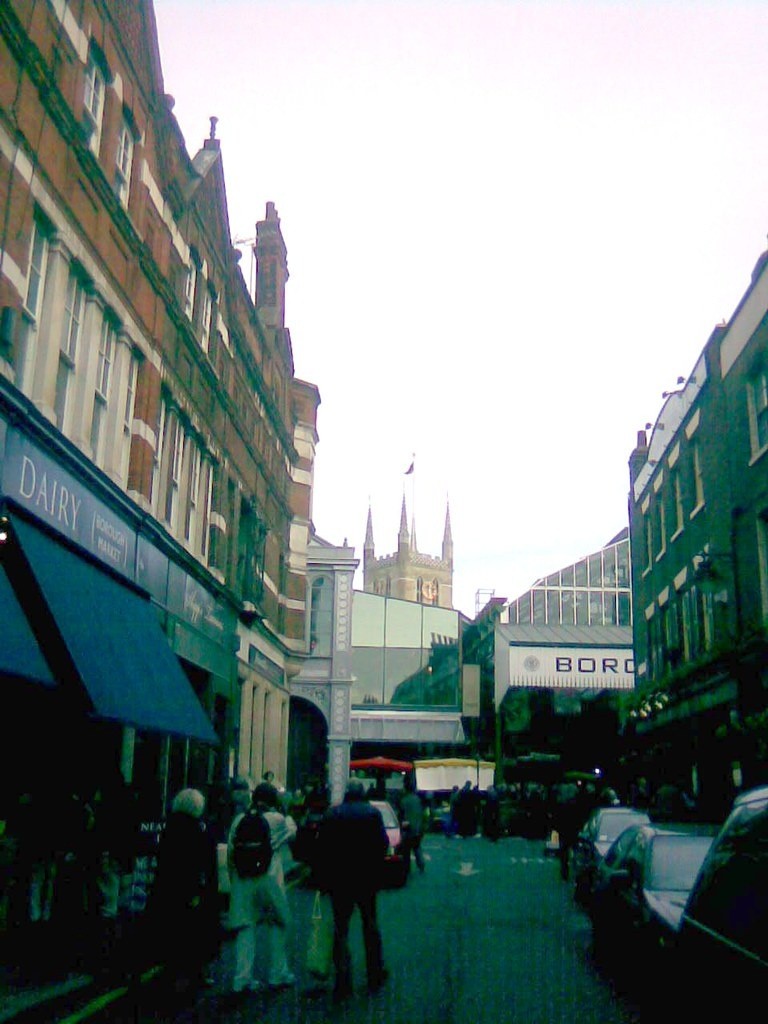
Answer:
[305,889,332,978]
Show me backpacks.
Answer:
[232,809,274,878]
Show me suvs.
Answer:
[669,788,768,1024]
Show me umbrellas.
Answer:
[349,757,413,790]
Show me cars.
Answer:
[330,798,410,880]
[588,822,720,1018]
[569,807,661,908]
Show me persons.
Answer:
[557,777,613,881]
[0,745,165,988]
[226,770,306,992]
[302,779,389,1002]
[397,775,429,876]
[145,790,212,1024]
[450,778,559,840]
[626,774,696,826]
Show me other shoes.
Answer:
[369,968,387,993]
[233,978,259,992]
[199,979,215,985]
[269,972,295,986]
[332,987,351,1003]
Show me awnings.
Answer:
[351,710,465,743]
[0,495,221,746]
